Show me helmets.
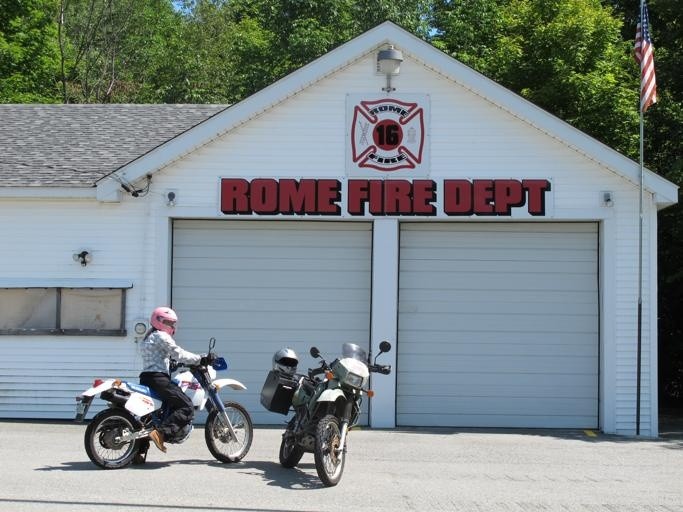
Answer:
[272,348,299,379]
[150,307,178,336]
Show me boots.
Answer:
[149,429,168,453]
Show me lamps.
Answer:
[600,190,615,207]
[166,190,177,208]
[73,250,94,268]
[376,47,404,93]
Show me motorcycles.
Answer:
[260,340,392,487]
[74,337,254,470]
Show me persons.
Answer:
[137,305,214,454]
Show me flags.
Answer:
[631,0,658,115]
